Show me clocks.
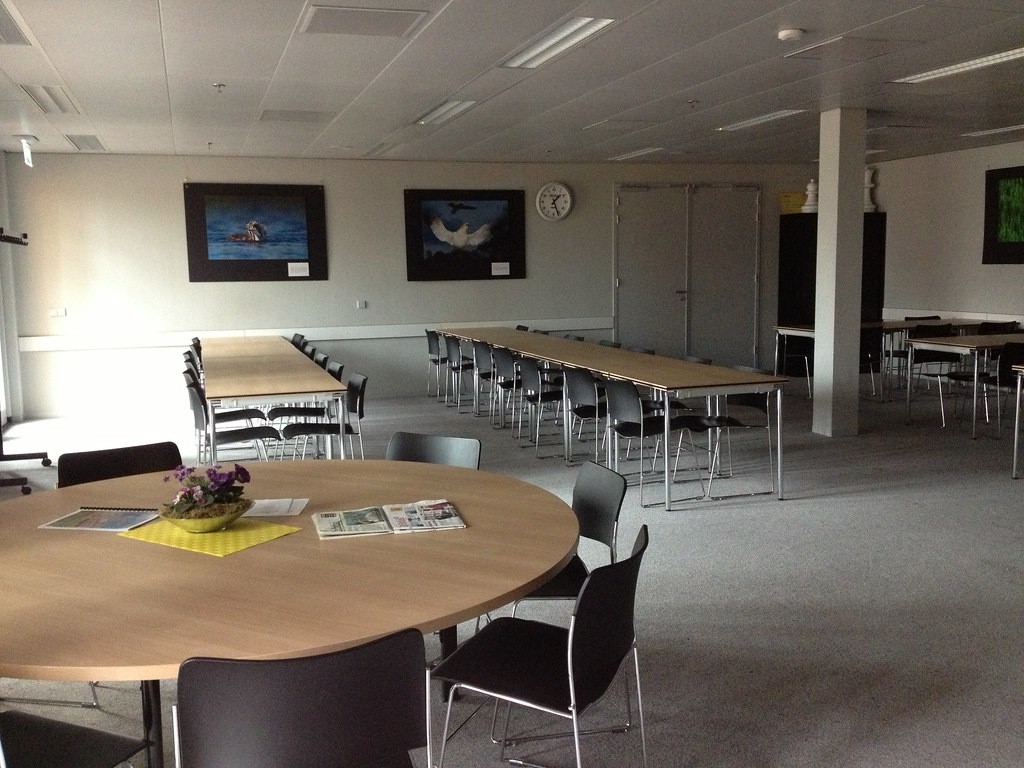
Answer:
[535,182,574,222]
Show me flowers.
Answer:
[161,463,252,513]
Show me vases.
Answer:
[158,498,256,534]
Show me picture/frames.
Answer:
[403,189,527,281]
[981,166,1024,265]
[182,181,329,282]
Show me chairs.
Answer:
[184,332,369,463]
[384,430,483,471]
[0,709,154,768]
[424,324,792,512]
[781,315,1024,442]
[171,627,432,768]
[472,458,628,639]
[56,441,185,489]
[429,523,652,768]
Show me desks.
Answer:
[0,457,582,768]
[198,318,1024,514]
